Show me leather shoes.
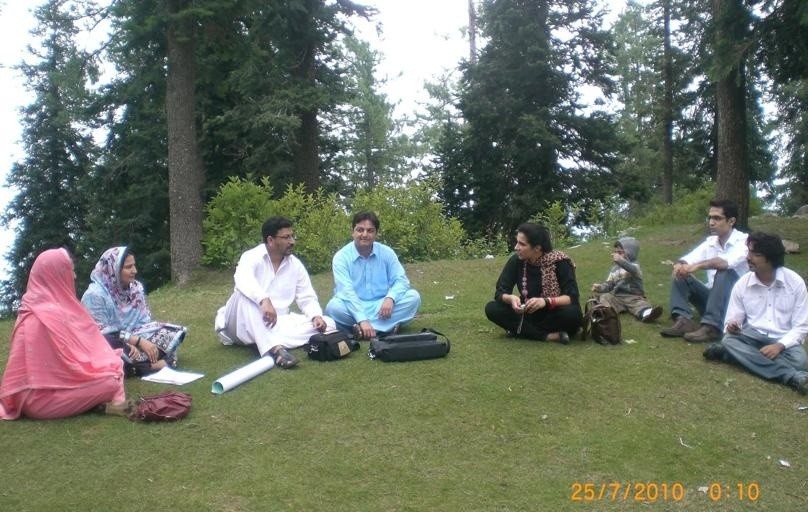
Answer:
[684,324,720,341]
[660,315,696,336]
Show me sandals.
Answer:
[352,322,364,340]
[383,323,399,335]
[560,329,569,343]
[106,400,138,416]
[642,305,663,321]
[269,347,300,367]
[160,356,177,369]
[127,361,152,376]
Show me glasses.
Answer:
[271,234,296,240]
[706,215,727,224]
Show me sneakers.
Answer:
[703,343,726,359]
[789,370,808,394]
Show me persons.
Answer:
[485,223,583,345]
[703,230,808,394]
[215,215,337,369]
[325,211,422,341]
[591,236,664,324]
[660,198,750,344]
[79,246,187,379]
[1,246,125,420]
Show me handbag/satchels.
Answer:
[137,389,192,420]
[307,332,352,361]
[582,299,621,345]
[369,327,451,362]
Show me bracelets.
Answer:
[135,336,141,347]
[543,297,556,311]
